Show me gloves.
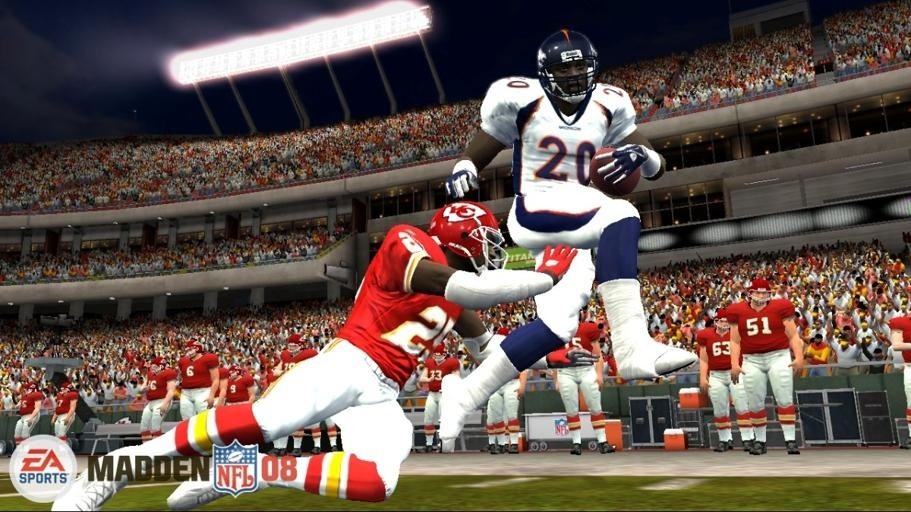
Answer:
[547,348,600,368]
[537,244,577,284]
[594,144,646,185]
[444,160,477,198]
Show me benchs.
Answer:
[402,411,486,453]
[89,421,188,460]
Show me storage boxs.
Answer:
[603,419,624,452]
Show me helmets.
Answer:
[714,309,730,332]
[428,201,509,272]
[150,357,166,374]
[185,340,205,360]
[24,383,36,390]
[538,29,600,103]
[287,335,305,352]
[61,383,72,389]
[746,280,772,305]
[228,366,242,377]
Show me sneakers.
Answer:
[743,440,767,454]
[600,441,615,454]
[786,438,799,454]
[571,443,581,454]
[167,444,267,510]
[426,445,432,452]
[51,446,137,511]
[487,445,518,454]
[714,440,733,451]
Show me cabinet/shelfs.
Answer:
[627,387,911,451]
